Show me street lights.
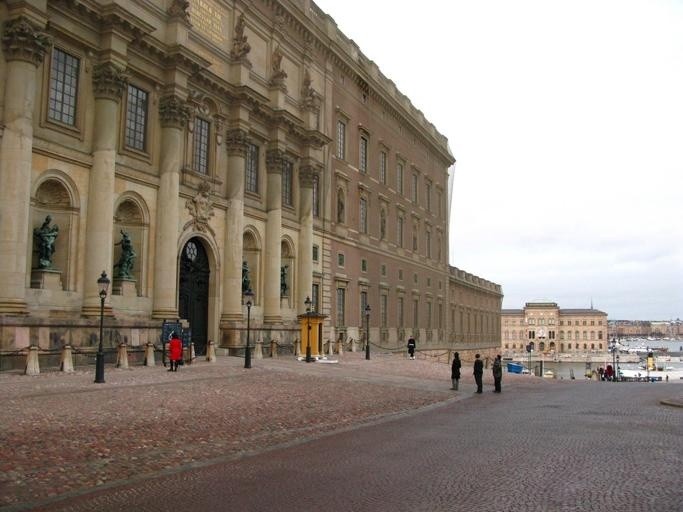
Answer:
[243,286,255,368]
[93,270,109,382]
[304,295,312,362]
[365,304,372,360]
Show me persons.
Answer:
[493,355,502,393]
[115,229,137,277]
[472,353,483,394]
[598,364,612,381]
[168,332,182,372]
[449,352,461,390]
[281,264,289,296]
[34,214,59,265]
[408,335,415,360]
[243,260,252,292]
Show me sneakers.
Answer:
[493,391,500,393]
[475,392,482,394]
[450,388,458,390]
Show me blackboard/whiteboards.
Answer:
[162,323,181,342]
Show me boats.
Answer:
[612,328,681,352]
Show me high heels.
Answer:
[168,369,173,371]
[174,368,177,371]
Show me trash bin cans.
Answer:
[507,362,522,372]
